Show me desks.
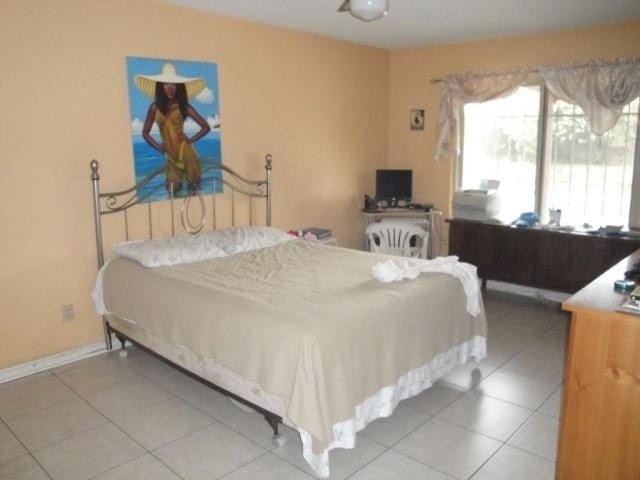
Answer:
[445,218,639,295]
[555,248,639,479]
[361,210,441,252]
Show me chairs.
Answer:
[365,222,431,258]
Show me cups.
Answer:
[549,208,561,226]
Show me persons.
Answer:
[134,64,212,197]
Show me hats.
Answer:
[134,64,205,99]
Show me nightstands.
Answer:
[321,237,337,247]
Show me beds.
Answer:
[90,154,488,480]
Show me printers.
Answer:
[451,179,502,221]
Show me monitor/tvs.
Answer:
[375,169,413,208]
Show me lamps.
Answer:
[337,0,389,22]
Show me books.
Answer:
[616,285,640,316]
[302,227,331,239]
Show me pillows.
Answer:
[112,225,298,268]
